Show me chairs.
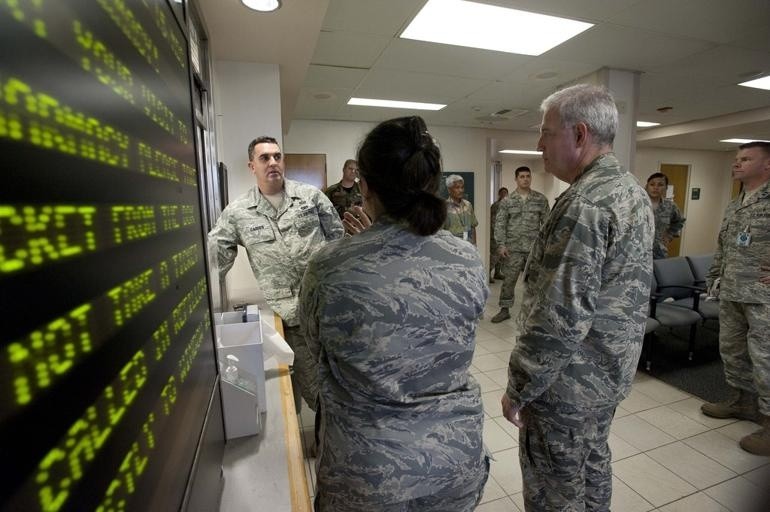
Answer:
[636,253,743,370]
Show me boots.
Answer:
[701,385,761,420]
[491,307,511,323]
[740,414,770,456]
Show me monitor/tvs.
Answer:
[1,1,226,512]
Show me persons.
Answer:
[207,136,344,459]
[491,166,552,323]
[491,187,508,282]
[702,143,770,457]
[502,83,655,512]
[646,172,685,260]
[324,160,371,235]
[299,116,491,512]
[443,175,477,249]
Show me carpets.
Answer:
[643,361,770,426]
